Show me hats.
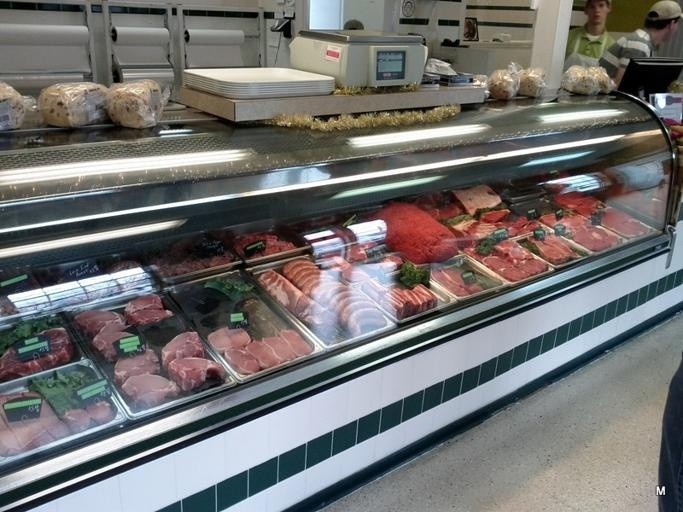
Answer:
[646,0,682,21]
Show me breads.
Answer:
[0,78,161,128]
[488,65,615,100]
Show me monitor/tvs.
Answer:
[616,55,683,103]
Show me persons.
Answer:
[563,0,616,72]
[599,0,683,85]
[659,363,681,512]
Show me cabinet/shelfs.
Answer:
[0,91,682,511]
